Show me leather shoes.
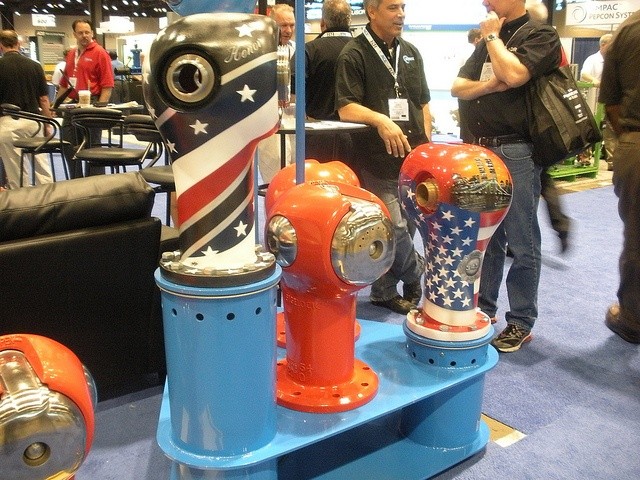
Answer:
[403,256,425,305]
[370,293,417,315]
[605,305,640,344]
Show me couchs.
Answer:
[1,171,168,403]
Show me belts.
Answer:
[474,136,532,147]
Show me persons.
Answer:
[289,1,353,169]
[1,30,55,188]
[60,19,114,183]
[448,0,562,352]
[520,0,576,263]
[581,29,619,174]
[336,0,442,315]
[52,44,72,91]
[108,50,125,80]
[599,7,639,346]
[268,3,297,120]
[458,25,485,64]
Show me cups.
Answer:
[79,90,91,106]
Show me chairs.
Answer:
[124,112,168,168]
[66,116,146,178]
[1,103,68,188]
[123,113,175,227]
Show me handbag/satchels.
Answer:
[526,24,602,166]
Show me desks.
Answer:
[49,102,139,183]
[256,115,371,203]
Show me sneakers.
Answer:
[491,324,532,353]
[608,162,613,170]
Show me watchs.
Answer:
[483,32,501,43]
